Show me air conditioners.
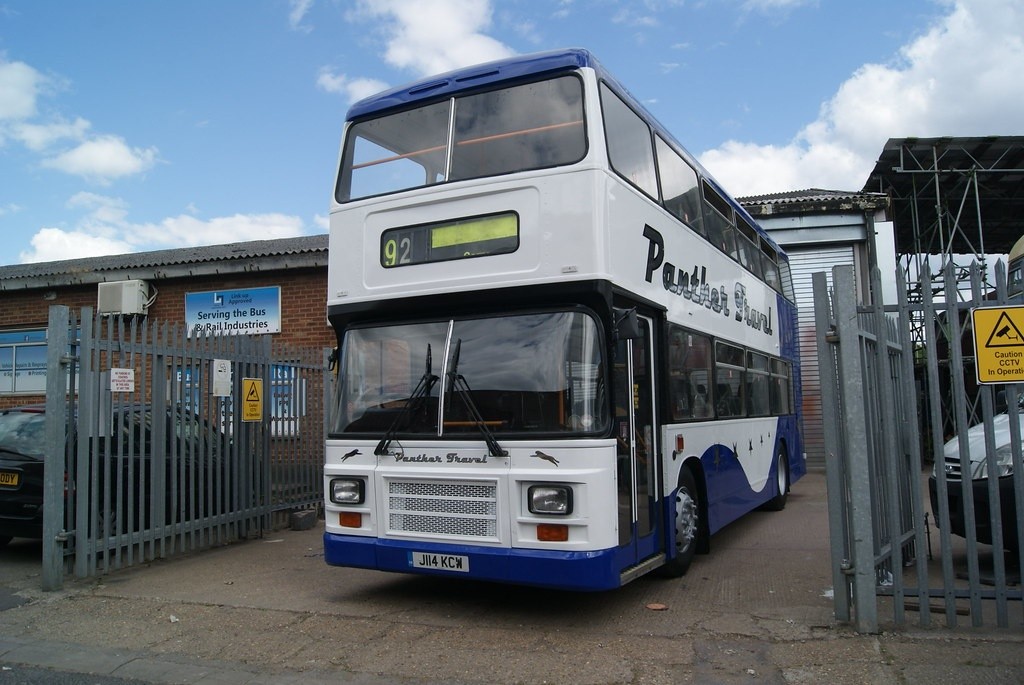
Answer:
[97,279,149,320]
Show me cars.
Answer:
[1,394,278,559]
[927,391,1024,549]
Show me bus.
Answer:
[322,47,806,594]
[1004,233,1024,304]
[920,288,1004,399]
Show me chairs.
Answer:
[573,378,782,428]
